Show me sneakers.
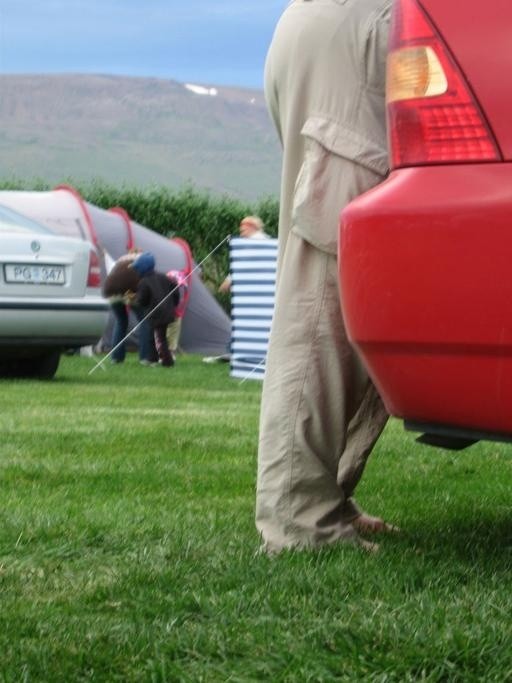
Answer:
[111,353,177,367]
[355,512,403,540]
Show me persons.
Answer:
[216,215,271,293]
[136,272,179,367]
[101,251,155,364]
[254,0,399,554]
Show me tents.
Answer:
[227,237,278,380]
[0,182,231,359]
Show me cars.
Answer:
[0,203,112,381]
[336,0,512,452]
